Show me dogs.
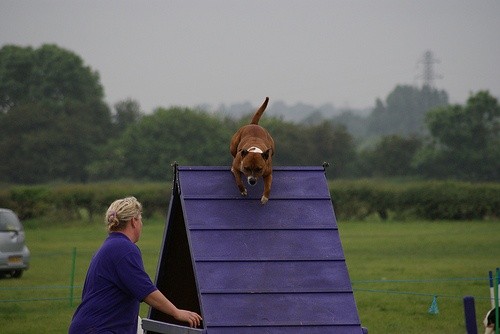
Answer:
[230,96,275,204]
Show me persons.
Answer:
[68,197,203,334]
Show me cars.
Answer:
[0,208,29,278]
